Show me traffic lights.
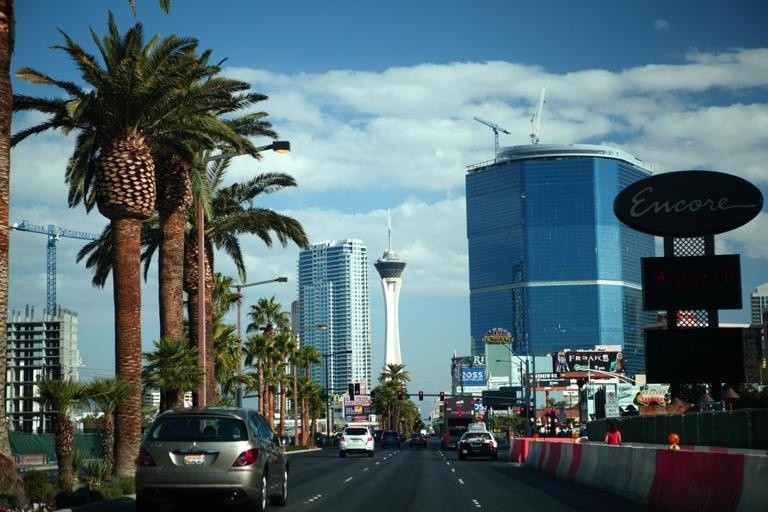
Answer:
[398,391,402,400]
[348,383,360,400]
[440,392,444,401]
[520,406,534,417]
[371,391,375,400]
[419,391,423,400]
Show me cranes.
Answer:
[474,115,512,159]
[10,219,101,315]
[530,87,545,144]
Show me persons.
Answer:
[604,421,623,445]
[610,352,626,375]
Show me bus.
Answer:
[441,398,475,450]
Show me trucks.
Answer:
[683,401,723,413]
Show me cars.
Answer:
[135,406,291,512]
[457,422,499,460]
[337,426,427,458]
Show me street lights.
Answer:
[198,141,290,407]
[275,324,328,349]
[321,350,352,449]
[496,360,536,432]
[229,277,288,408]
